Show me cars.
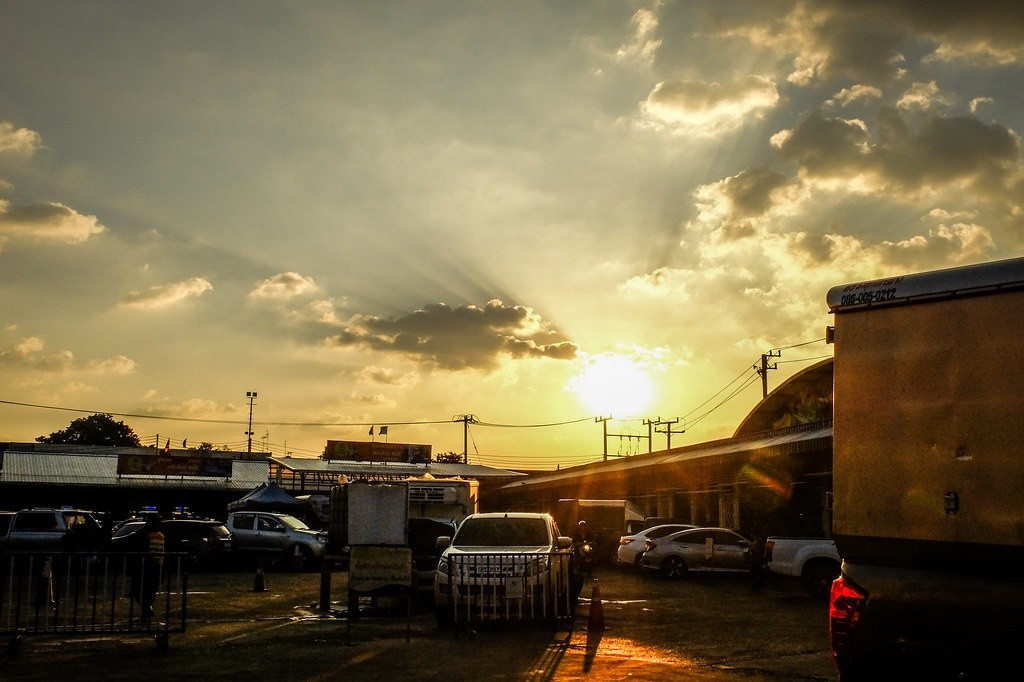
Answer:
[618,523,699,572]
[557,522,593,601]
[641,528,768,581]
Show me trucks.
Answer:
[829,258,1024,682]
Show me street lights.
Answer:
[245,391,258,460]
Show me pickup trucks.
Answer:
[766,536,842,593]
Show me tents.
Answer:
[227,481,308,519]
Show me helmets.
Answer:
[578,520,586,528]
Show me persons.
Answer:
[572,520,593,557]
[127,513,166,626]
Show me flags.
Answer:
[369,426,373,435]
[379,426,387,435]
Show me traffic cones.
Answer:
[580,578,612,633]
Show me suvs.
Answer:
[223,512,331,568]
[434,511,573,626]
[575,499,647,565]
[1,506,241,590]
